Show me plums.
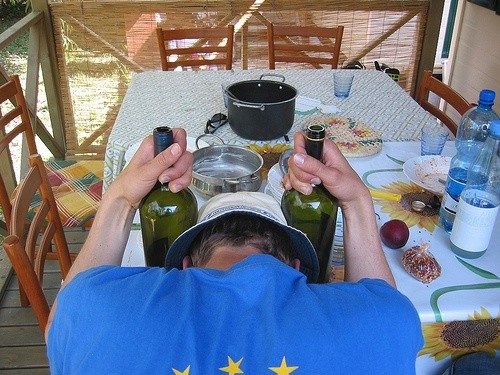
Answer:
[379,219,409,249]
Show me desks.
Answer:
[101,69,500,375]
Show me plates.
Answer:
[124,136,210,163]
[403,155,490,196]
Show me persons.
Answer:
[46,131,425,375]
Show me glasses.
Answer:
[203,112,229,135]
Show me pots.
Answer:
[221,73,299,140]
[191,134,264,197]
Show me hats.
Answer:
[165,190,322,284]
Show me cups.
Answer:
[420,125,449,155]
[334,71,354,97]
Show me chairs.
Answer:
[268,21,346,67]
[2,154,73,336]
[154,23,234,73]
[0,74,105,308]
[417,68,478,137]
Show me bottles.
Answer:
[437,90,500,233]
[281,124,338,284]
[139,125,198,267]
[448,119,500,259]
